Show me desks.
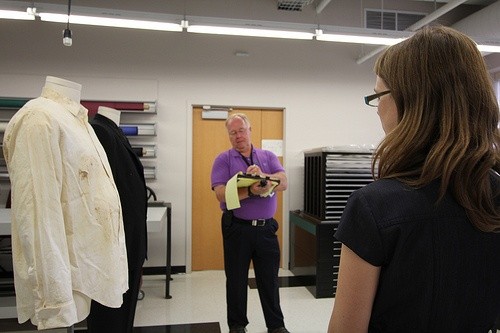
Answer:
[1,202,173,298]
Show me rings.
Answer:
[254,164,256,167]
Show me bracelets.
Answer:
[248,186,254,196]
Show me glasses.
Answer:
[364,90,391,107]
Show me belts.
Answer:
[234,218,273,227]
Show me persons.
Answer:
[210,112,290,333]
[2,74,130,330]
[326,21,500,333]
[88,106,149,330]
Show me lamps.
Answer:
[63,1,73,47]
